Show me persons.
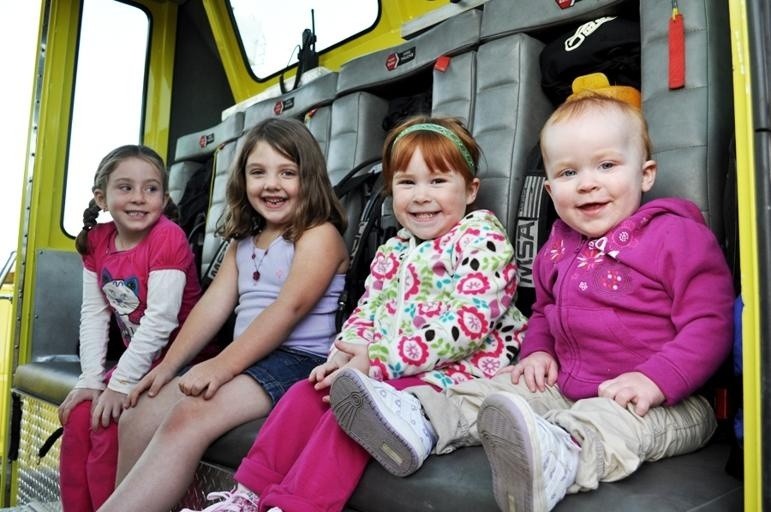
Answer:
[96,118,351,512]
[179,113,530,512]
[329,87,736,512]
[57,146,201,512]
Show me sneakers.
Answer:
[180,485,260,512]
[330,367,437,477]
[477,392,579,512]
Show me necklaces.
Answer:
[251,227,278,280]
